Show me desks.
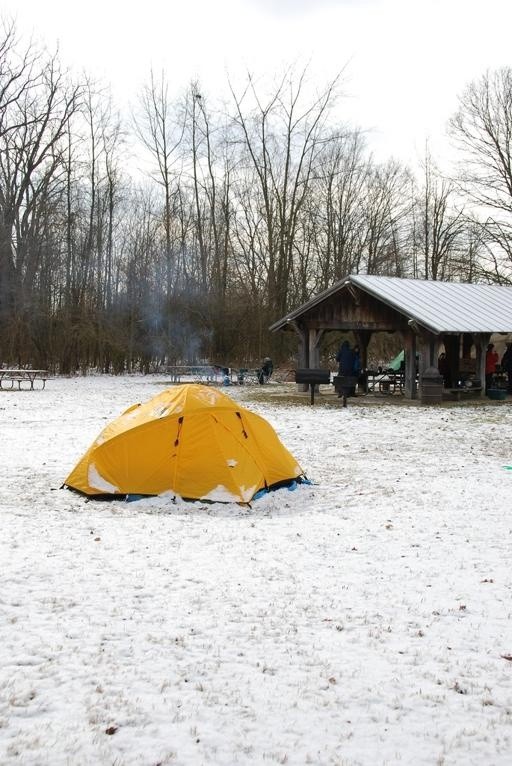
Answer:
[367,369,419,395]
[165,365,217,384]
[0,369,48,391]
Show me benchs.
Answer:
[166,372,196,376]
[6,376,47,382]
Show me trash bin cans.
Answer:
[422,367,445,404]
[222,368,231,386]
[240,369,248,380]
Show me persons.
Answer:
[335,339,361,398]
[501,342,512,395]
[256,357,273,385]
[438,352,449,375]
[486,343,499,396]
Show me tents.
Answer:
[59,383,309,510]
[387,349,423,370]
